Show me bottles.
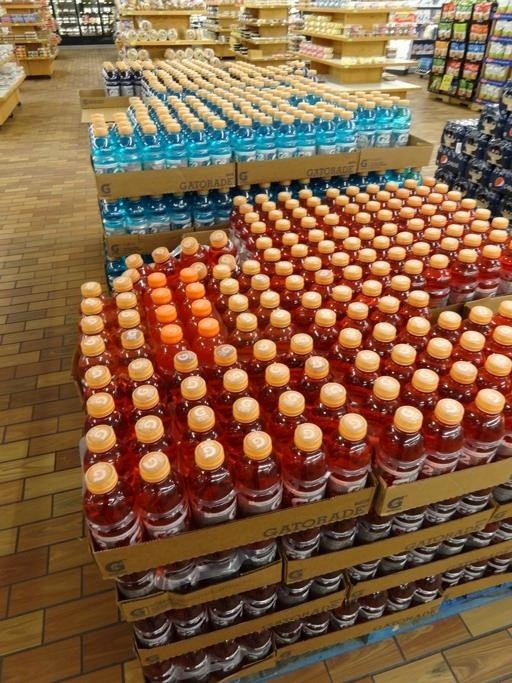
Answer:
[433,60,512,217]
[75,175,511,682]
[74,60,426,282]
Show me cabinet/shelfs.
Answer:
[0,0,512,682]
